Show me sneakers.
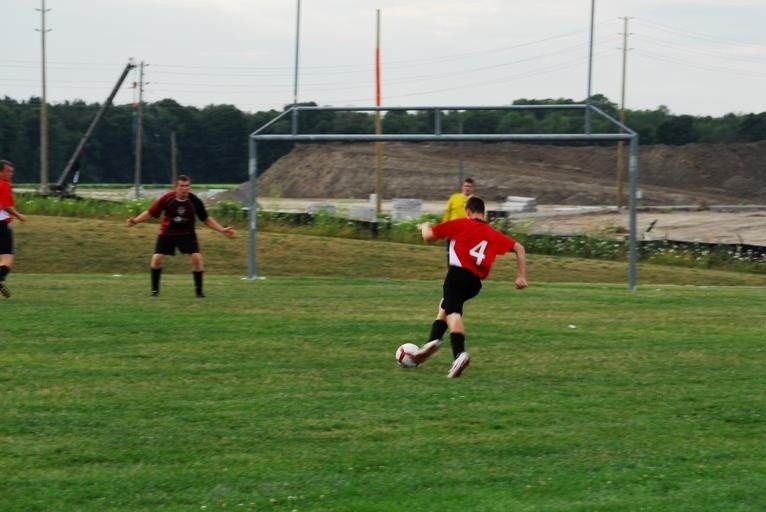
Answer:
[413,340,442,364]
[448,352,470,378]
[0,280,13,298]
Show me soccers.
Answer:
[396,344,421,367]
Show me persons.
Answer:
[410,197,527,379]
[445,178,485,253]
[126,175,236,297]
[0,159,26,298]
